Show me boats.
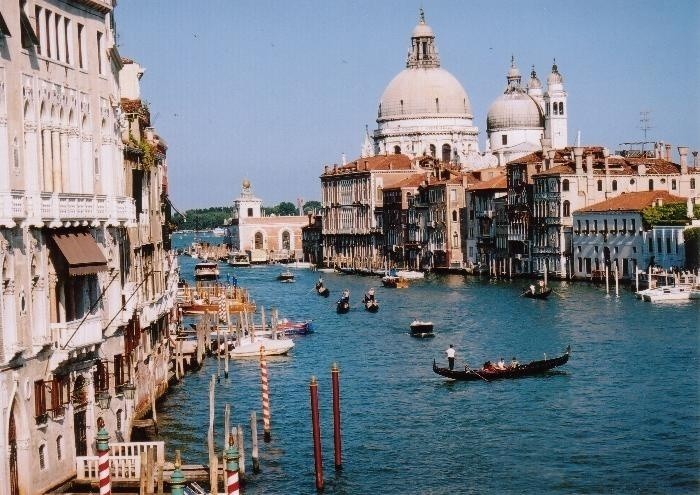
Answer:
[433,343,571,381]
[409,323,434,338]
[316,283,379,313]
[280,273,295,282]
[195,252,250,280]
[524,288,552,299]
[227,336,295,357]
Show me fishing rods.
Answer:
[64,271,120,348]
[102,269,156,333]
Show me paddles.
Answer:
[454,358,491,383]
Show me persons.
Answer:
[481,356,519,374]
[445,344,456,371]
[337,288,351,304]
[538,279,544,293]
[319,275,325,288]
[368,288,376,301]
[530,285,536,294]
